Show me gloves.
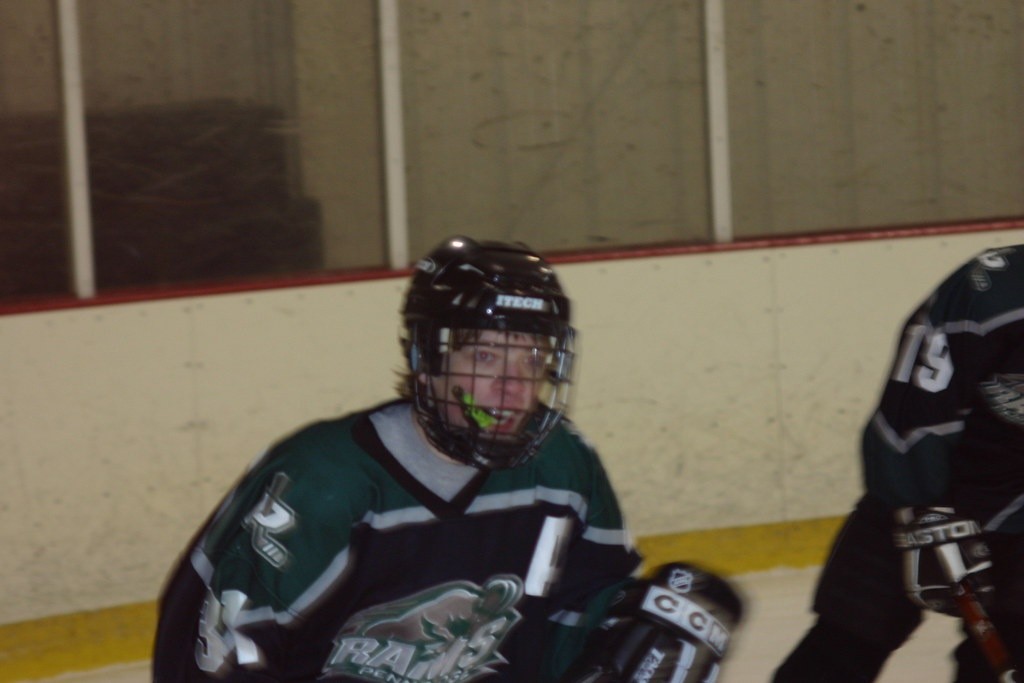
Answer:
[894,503,995,618]
[567,562,743,683]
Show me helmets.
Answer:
[400,235,577,475]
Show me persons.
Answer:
[767,242,1024,683]
[152,234,743,683]
